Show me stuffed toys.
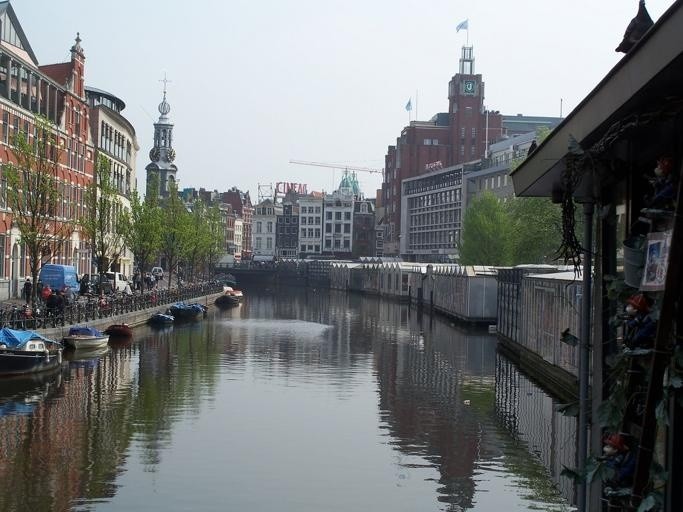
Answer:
[624,292,654,350]
[602,433,634,490]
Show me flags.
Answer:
[405,98,412,111]
[456,20,468,32]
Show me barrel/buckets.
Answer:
[622,215,652,289]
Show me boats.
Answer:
[151,303,208,324]
[63,322,132,350]
[216,294,239,306]
[0,328,64,375]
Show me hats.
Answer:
[26,279,42,281]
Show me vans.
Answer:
[38,264,132,293]
[151,267,164,279]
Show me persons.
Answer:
[23,277,69,328]
[132,270,156,291]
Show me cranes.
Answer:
[288,159,384,184]
